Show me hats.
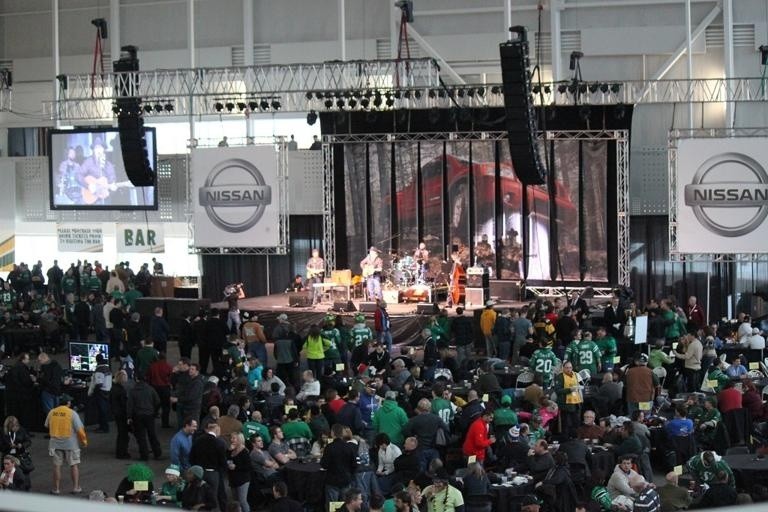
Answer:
[355,313,364,322]
[190,466,203,479]
[277,314,287,320]
[385,391,395,399]
[501,395,511,404]
[324,314,336,321]
[59,395,73,403]
[166,465,180,476]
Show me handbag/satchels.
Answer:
[18,452,34,472]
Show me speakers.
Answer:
[416,304,438,314]
[499,40,545,186]
[107,57,158,187]
[289,295,310,306]
[358,301,380,312]
[334,300,356,311]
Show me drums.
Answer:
[389,269,412,286]
[404,255,413,266]
[419,262,430,273]
[394,264,405,271]
[64,172,84,200]
[482,254,495,267]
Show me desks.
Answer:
[3,325,40,358]
[546,441,615,483]
[718,451,768,492]
[586,341,768,473]
[479,469,540,509]
[282,455,324,500]
[440,359,529,401]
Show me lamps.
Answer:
[212,95,282,112]
[110,99,176,116]
[305,85,501,109]
[530,81,622,95]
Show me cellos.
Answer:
[451,257,466,304]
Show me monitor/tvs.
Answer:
[67,341,110,375]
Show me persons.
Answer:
[0,238,767,512]
[108,135,137,205]
[74,145,88,166]
[501,228,523,278]
[505,192,517,216]
[76,137,116,204]
[475,231,495,272]
[452,234,469,261]
[57,147,81,189]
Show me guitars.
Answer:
[81,174,134,205]
[506,246,537,261]
[306,268,327,280]
[362,264,382,277]
